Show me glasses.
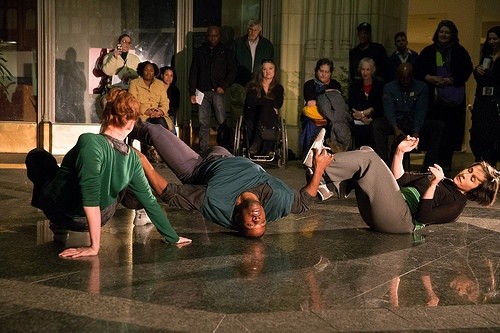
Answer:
[121,39,132,44]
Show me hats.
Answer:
[356,20,372,32]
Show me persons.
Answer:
[242,59,285,156]
[303,59,342,158]
[415,20,473,173]
[387,33,419,84]
[127,121,334,239]
[24,86,192,258]
[350,23,389,80]
[188,25,236,159]
[12,84,37,123]
[227,18,274,130]
[76,239,499,311]
[158,66,180,124]
[347,57,383,150]
[102,35,142,91]
[56,46,86,122]
[472,26,500,169]
[381,63,429,172]
[302,127,500,235]
[127,61,174,163]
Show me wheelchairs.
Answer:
[234,115,288,168]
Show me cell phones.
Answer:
[116,44,123,55]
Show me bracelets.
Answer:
[361,111,365,117]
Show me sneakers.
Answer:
[302,126,334,201]
[132,208,152,226]
